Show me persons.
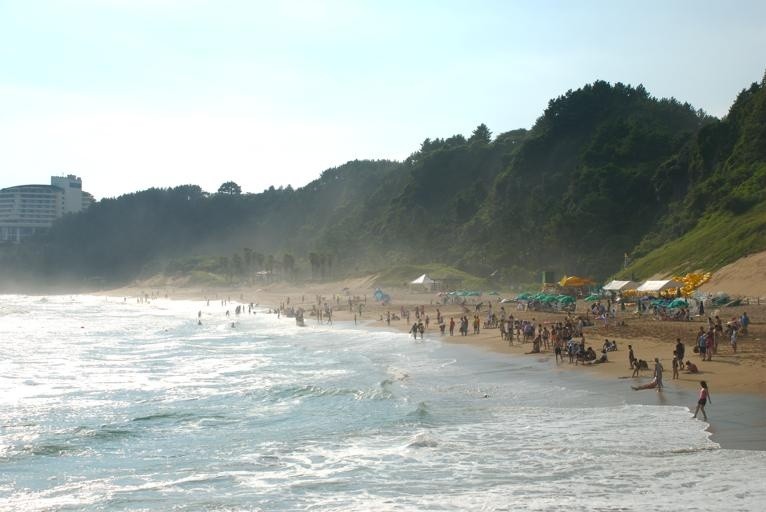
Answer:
[198,296,258,328]
[269,294,362,325]
[699,300,704,316]
[124,288,168,304]
[691,381,711,421]
[498,300,627,366]
[379,295,506,340]
[627,311,751,392]
[651,306,691,319]
